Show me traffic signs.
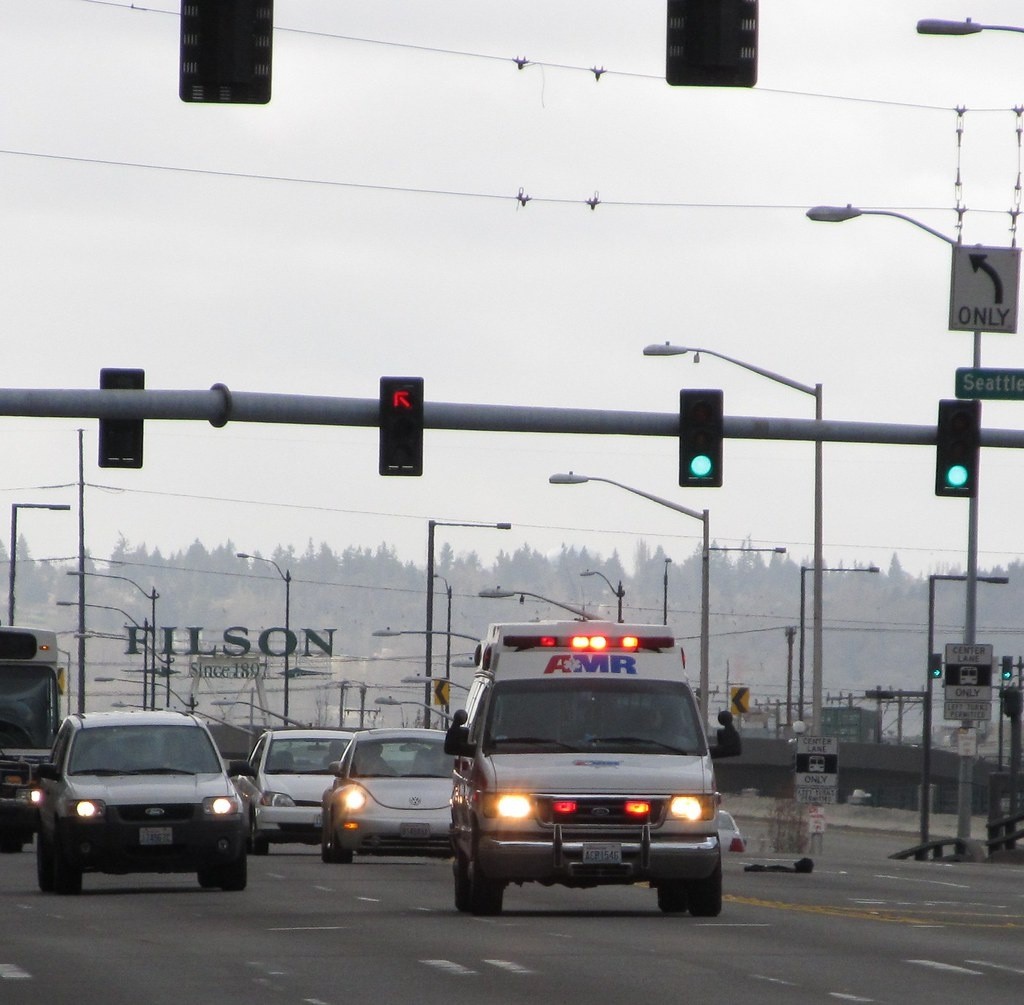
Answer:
[944,642,992,721]
[949,245,1020,334]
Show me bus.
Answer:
[0,625,64,851]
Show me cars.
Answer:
[236,728,458,863]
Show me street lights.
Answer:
[57,569,172,710]
[921,575,1009,844]
[808,207,979,852]
[581,569,625,623]
[643,343,823,853]
[8,503,70,625]
[237,553,291,726]
[424,520,511,728]
[799,566,880,721]
[549,473,709,736]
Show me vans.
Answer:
[443,621,743,916]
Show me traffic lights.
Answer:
[932,653,942,679]
[1002,655,1013,680]
[679,389,724,487]
[935,399,981,498]
[380,376,423,477]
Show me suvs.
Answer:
[36,710,249,895]
